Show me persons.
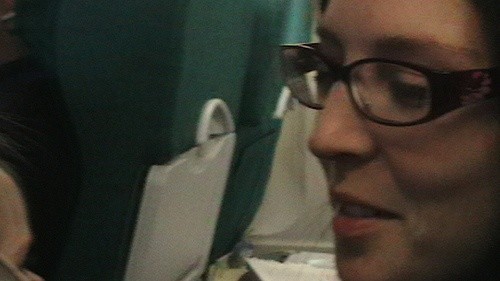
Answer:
[0,0,82,281]
[278,0,500,281]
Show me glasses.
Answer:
[279,43,500,129]
